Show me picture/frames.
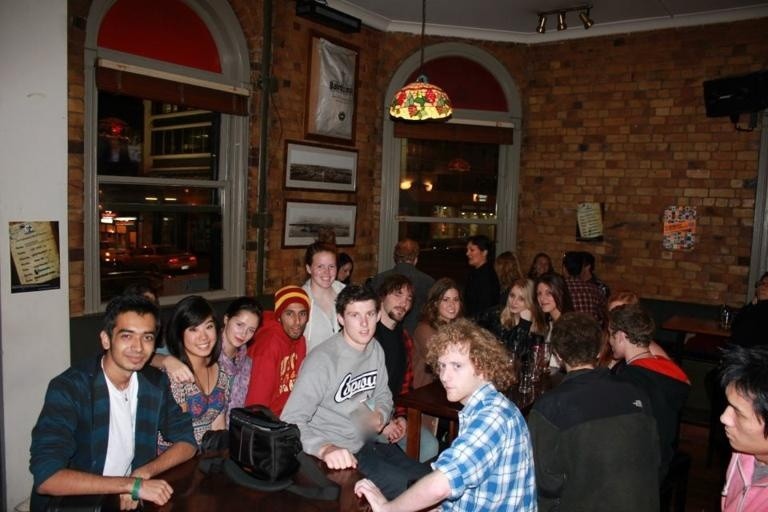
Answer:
[282,140,360,195]
[303,34,361,147]
[282,199,357,248]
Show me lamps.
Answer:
[391,0,453,123]
[536,4,594,34]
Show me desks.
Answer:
[398,363,568,462]
[141,443,381,510]
[661,314,731,359]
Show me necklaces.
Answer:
[193,367,210,406]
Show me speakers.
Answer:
[703,70,768,118]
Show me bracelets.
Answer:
[132,478,142,500]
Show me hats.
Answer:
[274,283,311,324]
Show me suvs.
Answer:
[99,241,130,266]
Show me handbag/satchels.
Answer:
[227,403,341,502]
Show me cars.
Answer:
[111,243,197,276]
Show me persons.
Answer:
[719,348,768,512]
[729,273,767,350]
[157,297,230,457]
[150,297,263,430]
[29,293,198,512]
[244,285,311,418]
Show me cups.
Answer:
[721,308,734,329]
[509,341,553,393]
